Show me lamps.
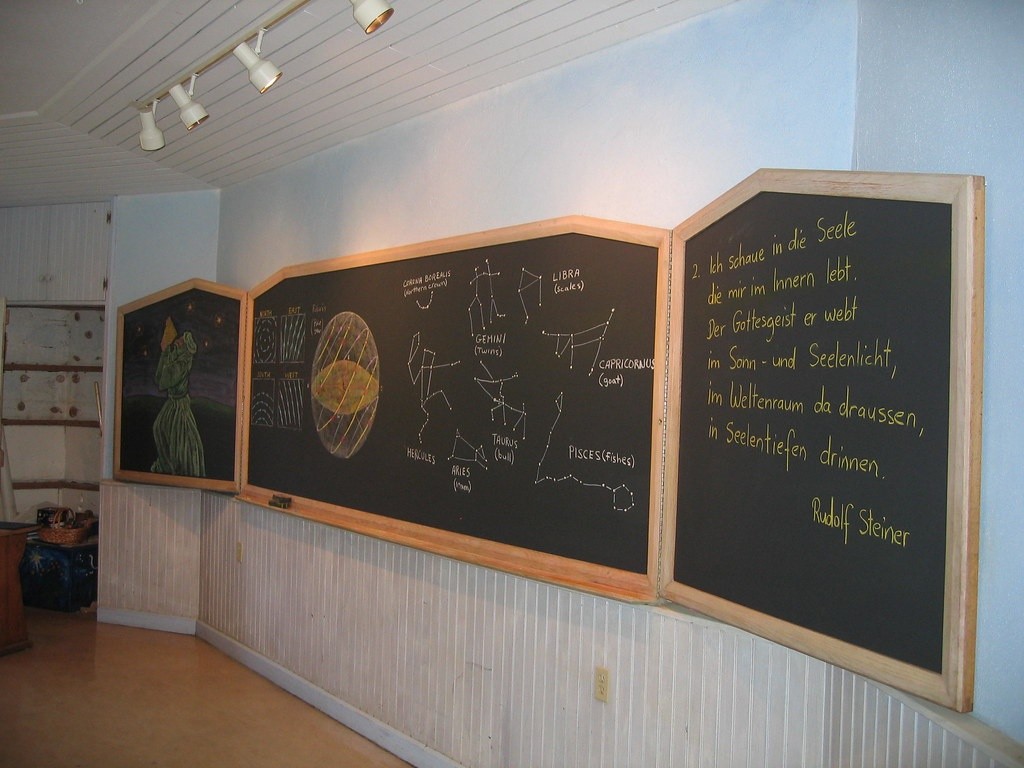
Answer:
[348,0,394,35]
[167,73,210,133]
[232,27,284,95]
[137,98,167,152]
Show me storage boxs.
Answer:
[18,535,97,613]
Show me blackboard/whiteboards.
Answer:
[111,271,247,495]
[242,214,671,605]
[659,169,985,714]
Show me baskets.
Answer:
[37,507,94,545]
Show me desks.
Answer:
[0,520,43,657]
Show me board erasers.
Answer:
[268,493,291,509]
